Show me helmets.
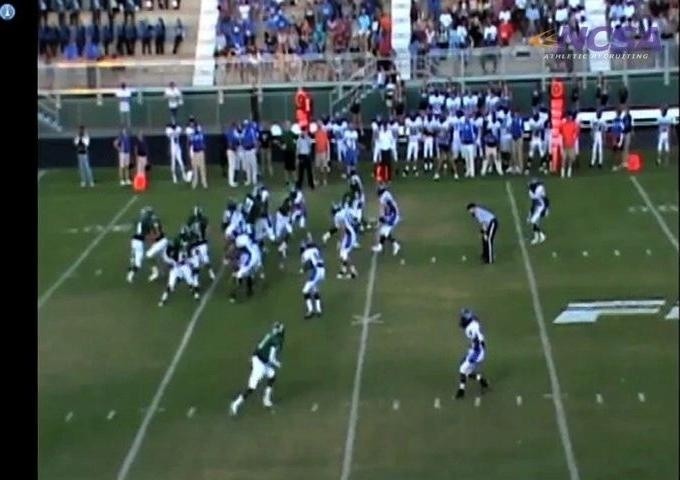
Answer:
[141,207,153,219]
[527,177,538,187]
[659,102,669,113]
[377,186,386,195]
[230,228,240,240]
[192,205,201,216]
[300,239,311,249]
[330,202,341,216]
[272,320,284,336]
[168,115,177,128]
[226,197,238,211]
[461,308,472,321]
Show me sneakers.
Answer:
[304,310,315,319]
[392,244,400,256]
[314,311,323,316]
[346,271,360,279]
[372,246,382,252]
[263,397,274,408]
[336,271,347,278]
[232,402,239,416]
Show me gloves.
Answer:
[300,268,303,274]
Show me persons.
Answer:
[126,205,216,308]
[38,0,185,57]
[164,80,183,126]
[215,0,390,81]
[225,114,346,188]
[410,1,590,79]
[116,81,132,133]
[653,102,677,165]
[183,117,201,176]
[189,124,208,190]
[324,86,373,282]
[289,189,314,244]
[133,129,148,174]
[467,202,498,265]
[230,320,286,416]
[527,178,549,246]
[164,123,187,182]
[371,73,635,182]
[606,0,680,54]
[113,127,133,186]
[222,185,293,306]
[454,308,489,399]
[298,240,326,319]
[74,125,95,188]
[370,184,402,256]
